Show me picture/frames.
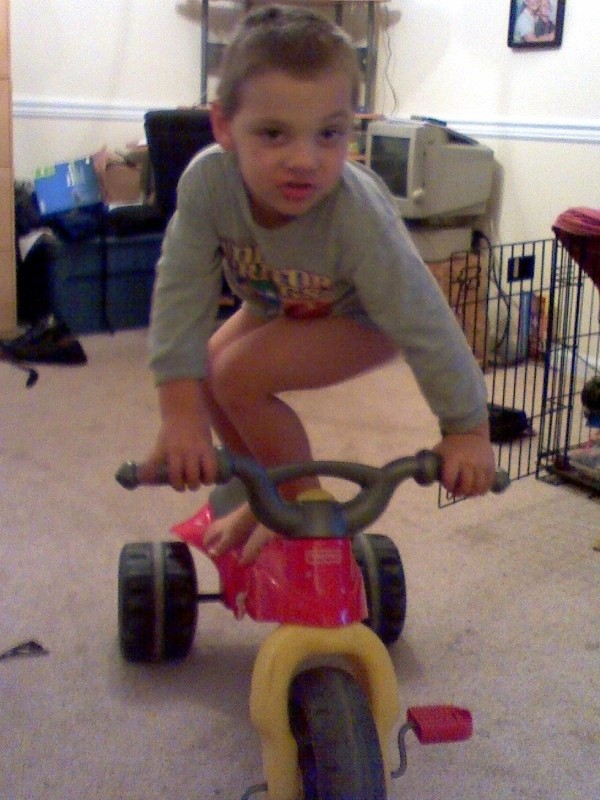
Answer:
[507,0,565,48]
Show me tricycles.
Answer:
[112,449,514,800]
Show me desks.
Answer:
[425,236,491,368]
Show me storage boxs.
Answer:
[46,233,163,334]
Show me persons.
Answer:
[144,1,496,563]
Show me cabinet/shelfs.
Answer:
[200,0,376,169]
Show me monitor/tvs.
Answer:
[364,121,495,227]
